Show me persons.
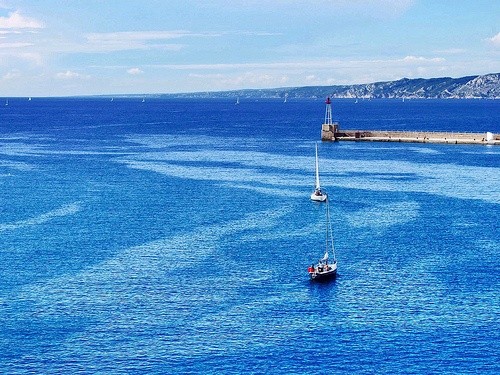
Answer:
[308,261,329,272]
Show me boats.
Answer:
[308,192,337,282]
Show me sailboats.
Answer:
[355,98,358,103]
[282,96,286,102]
[235,96,239,104]
[402,97,404,102]
[140,97,145,102]
[109,97,113,102]
[310,143,327,203]
[4,100,9,105]
[28,96,32,101]
